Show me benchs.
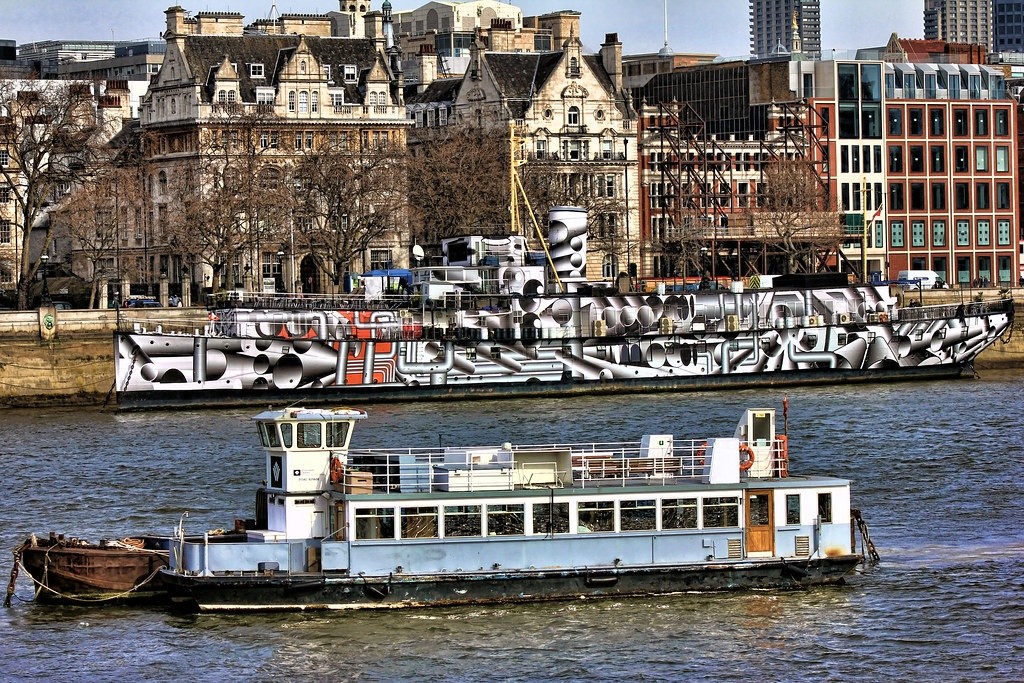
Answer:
[586,458,683,488]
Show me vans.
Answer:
[897,270,949,291]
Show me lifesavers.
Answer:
[738,444,756,471]
[697,442,707,465]
[330,457,342,483]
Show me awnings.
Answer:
[871,278,922,287]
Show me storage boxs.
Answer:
[336,471,373,494]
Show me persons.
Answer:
[908,298,923,307]
[134,298,144,307]
[1019,276,1024,287]
[111,291,121,308]
[972,274,990,288]
[123,299,132,307]
[169,295,182,306]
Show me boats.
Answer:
[20,519,256,606]
[159,393,881,613]
[113,117,1013,412]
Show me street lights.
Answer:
[39,253,52,307]
[275,249,286,295]
[699,245,711,292]
[877,189,897,281]
[609,230,615,288]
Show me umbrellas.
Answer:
[361,268,413,295]
